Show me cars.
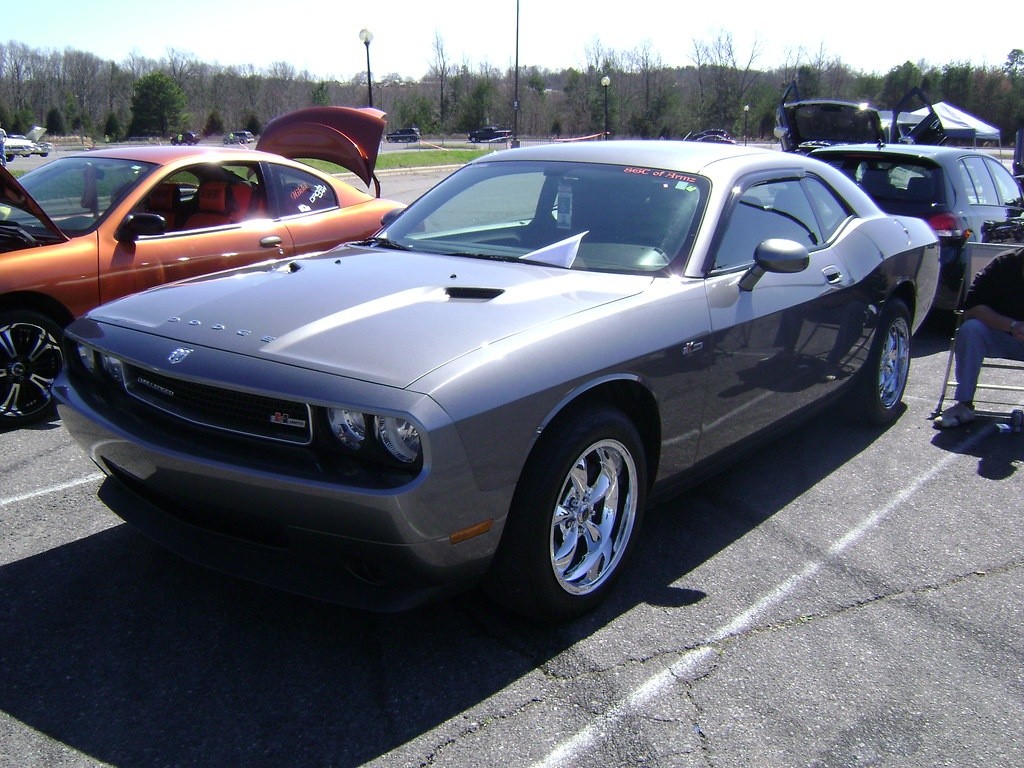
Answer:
[223,130,255,144]
[12,126,52,157]
[773,81,1024,329]
[0,106,410,427]
[693,127,734,139]
[51,139,941,622]
[697,135,736,145]
[171,130,201,145]
[0,128,32,162]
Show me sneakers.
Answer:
[933,403,975,426]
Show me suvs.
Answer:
[386,128,421,143]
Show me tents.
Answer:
[897,101,1002,163]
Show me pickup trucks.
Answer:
[468,125,512,143]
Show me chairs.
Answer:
[908,177,931,190]
[861,168,896,198]
[180,181,232,231]
[147,184,182,232]
[930,241,1024,424]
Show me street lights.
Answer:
[359,29,373,107]
[744,106,749,146]
[601,76,610,140]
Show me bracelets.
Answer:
[1009,320,1018,334]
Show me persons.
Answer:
[105,135,110,143]
[228,132,234,144]
[932,248,1024,426]
[83,134,89,151]
[0,122,7,168]
[756,264,889,381]
[177,134,183,145]
